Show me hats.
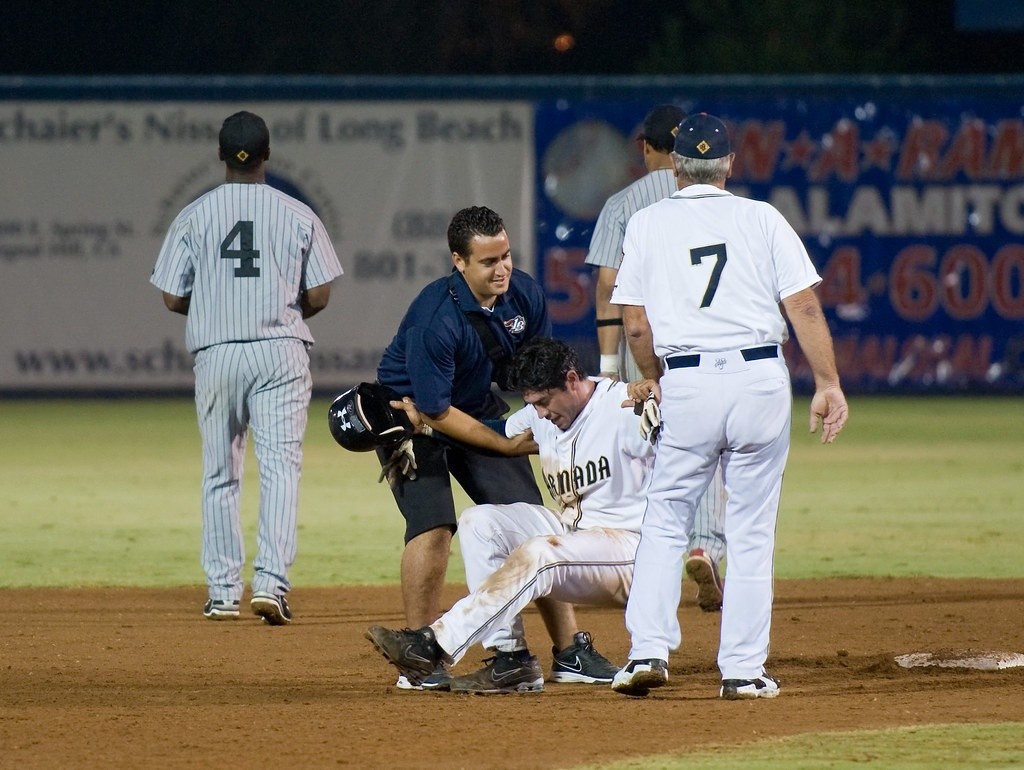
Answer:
[219,111,270,166]
[635,104,687,141]
[674,113,731,160]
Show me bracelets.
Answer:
[600,354,619,372]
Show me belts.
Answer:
[665,344,778,370]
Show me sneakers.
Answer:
[611,659,668,696]
[450,647,544,693]
[550,631,622,683]
[202,598,240,618]
[251,591,292,624]
[366,625,439,687]
[397,661,452,690]
[719,670,781,700]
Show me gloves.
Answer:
[633,392,661,446]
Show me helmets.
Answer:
[328,382,409,452]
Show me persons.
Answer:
[370,206,625,690]
[365,337,661,693]
[149,110,345,623]
[607,111,849,699]
[583,106,726,611]
